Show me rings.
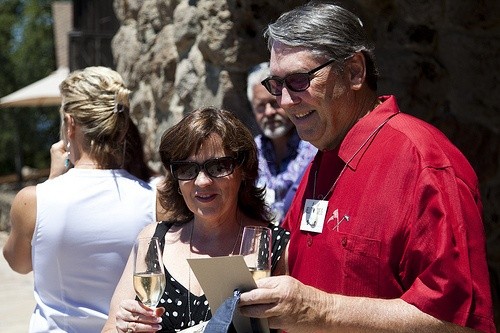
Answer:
[125,322,128,332]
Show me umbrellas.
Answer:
[0,65,70,106]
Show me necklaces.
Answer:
[75,162,98,165]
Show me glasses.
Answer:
[170,156,243,180]
[261,55,345,96]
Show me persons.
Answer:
[101,105,291,333]
[234,2,497,333]
[245,62,318,226]
[3,67,158,333]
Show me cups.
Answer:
[239,226,272,281]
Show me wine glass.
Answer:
[133,236,166,316]
